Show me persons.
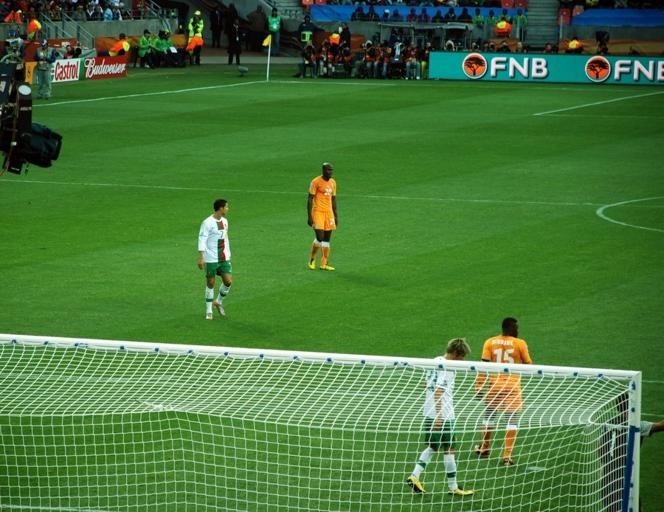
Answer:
[407,338,473,495]
[306,161,339,272]
[543,1,608,56]
[197,199,234,320]
[293,1,529,80]
[209,2,283,65]
[594,392,664,511]
[470,318,534,465]
[2,1,204,97]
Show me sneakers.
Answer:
[308,255,335,271]
[206,300,226,320]
[407,444,514,496]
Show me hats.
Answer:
[193,11,201,16]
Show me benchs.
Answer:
[303,61,422,81]
[133,45,189,68]
